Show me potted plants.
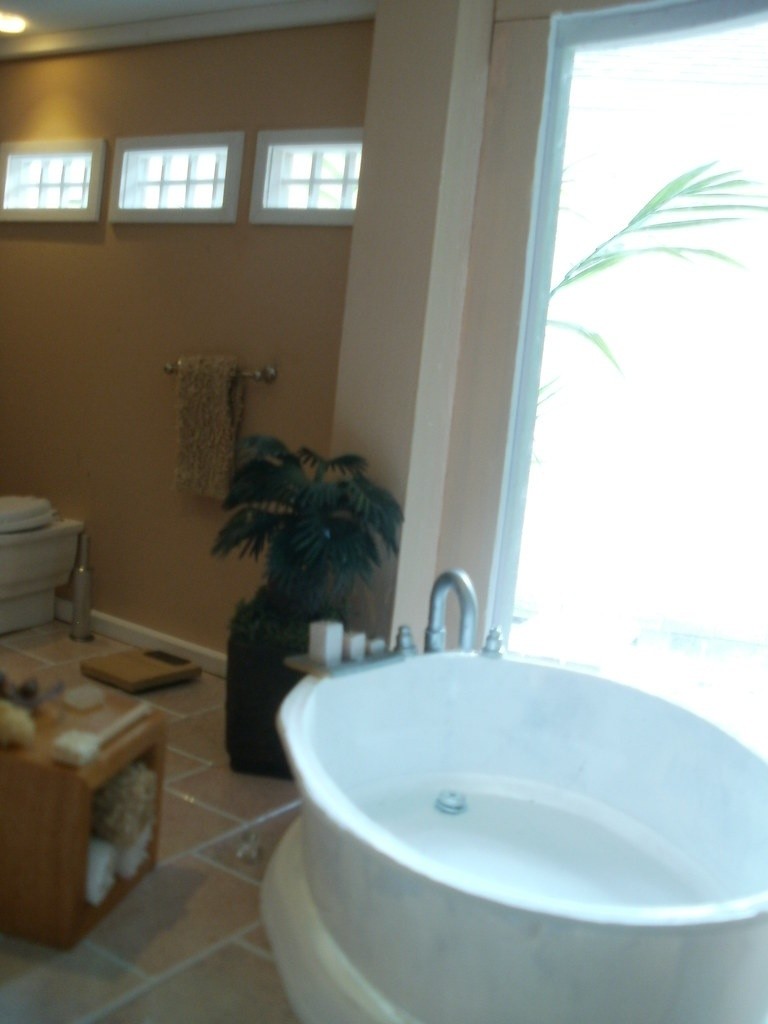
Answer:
[210,434,405,777]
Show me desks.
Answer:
[0,683,166,953]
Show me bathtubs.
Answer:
[265,649,767,1024]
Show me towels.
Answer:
[170,349,248,502]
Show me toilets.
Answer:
[0,491,83,633]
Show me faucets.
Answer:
[425,571,475,654]
